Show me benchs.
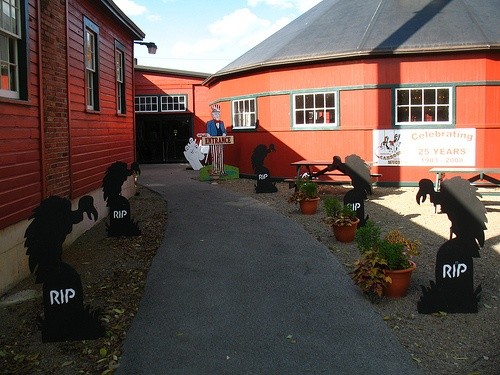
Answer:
[428,167,500,213]
[284,159,383,196]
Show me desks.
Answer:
[291,160,378,175]
[429,167,500,192]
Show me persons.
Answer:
[206,109,227,136]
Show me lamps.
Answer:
[134,42,158,55]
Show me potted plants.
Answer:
[287,172,321,215]
[319,196,360,243]
[348,219,418,303]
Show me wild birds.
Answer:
[250,143,276,175]
[101,159,142,208]
[327,153,374,200]
[415,176,488,258]
[23,194,99,285]
